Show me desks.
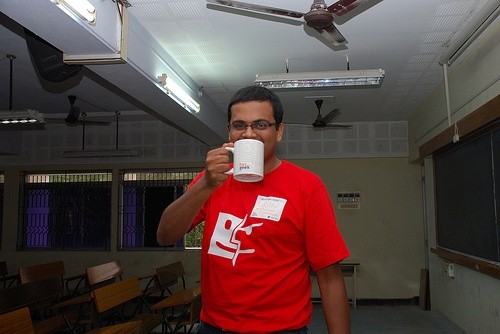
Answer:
[311,261,360,312]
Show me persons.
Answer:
[157,86,352,334]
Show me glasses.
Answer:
[229,121,276,130]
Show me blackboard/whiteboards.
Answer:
[433,118,500,262]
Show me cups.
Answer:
[224,139,264,181]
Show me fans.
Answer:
[281,99,354,129]
[44,94,112,127]
[205,0,370,47]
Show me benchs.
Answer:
[0,259,201,334]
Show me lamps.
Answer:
[157,72,202,114]
[254,54,387,91]
[61,0,98,26]
[438,0,500,68]
[0,53,46,126]
